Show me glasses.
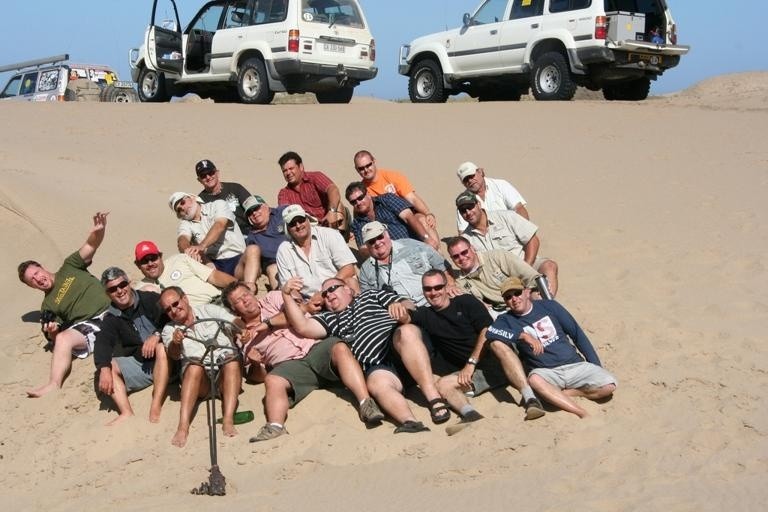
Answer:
[368,235,384,245]
[322,285,344,299]
[350,194,366,205]
[164,298,181,313]
[107,281,128,293]
[463,174,475,183]
[422,285,444,291]
[459,203,475,214]
[358,162,372,171]
[288,217,305,227]
[451,248,469,259]
[200,172,215,179]
[178,200,185,213]
[246,205,262,217]
[506,290,522,300]
[140,255,158,265]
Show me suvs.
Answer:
[1,54,139,102]
[397,0,691,101]
[128,0,379,102]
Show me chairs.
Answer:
[204,12,246,65]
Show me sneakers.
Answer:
[445,410,484,436]
[360,399,384,422]
[250,423,288,442]
[524,398,545,420]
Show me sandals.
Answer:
[428,398,450,425]
[394,420,430,433]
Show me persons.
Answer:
[18,151,617,449]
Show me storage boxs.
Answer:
[605,10,646,42]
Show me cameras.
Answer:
[382,283,398,294]
[41,310,56,322]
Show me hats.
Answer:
[135,241,159,261]
[362,221,386,244]
[242,195,265,214]
[196,160,215,175]
[457,162,478,181]
[168,192,186,211]
[455,190,476,209]
[501,277,524,296]
[282,203,305,223]
